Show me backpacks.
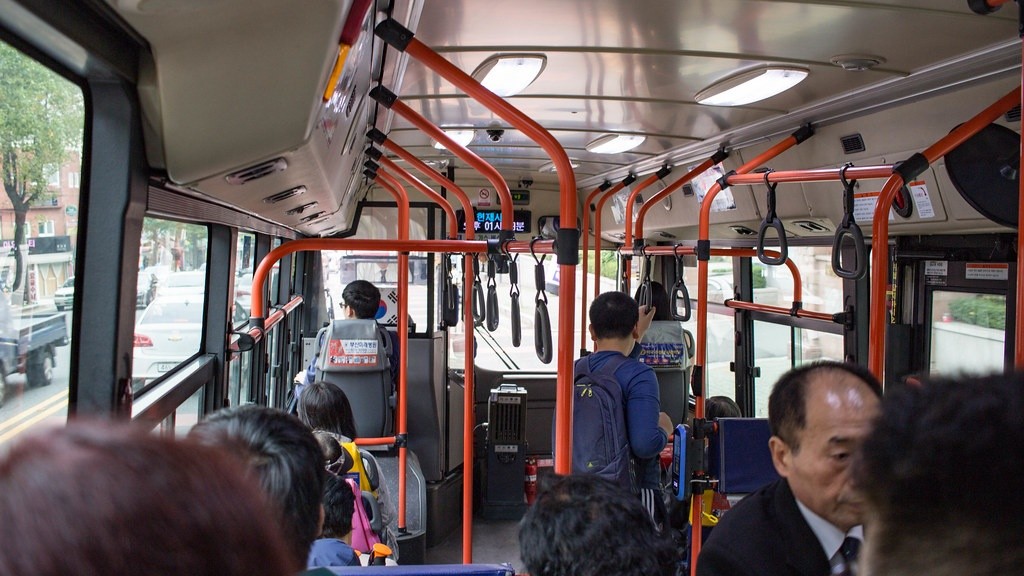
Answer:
[549,355,642,496]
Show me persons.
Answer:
[695,360,883,576]
[667,396,742,481]
[185,404,336,576]
[308,474,397,567]
[849,371,1024,576]
[634,281,672,320]
[0,419,301,576]
[297,382,400,562]
[314,432,354,474]
[294,280,400,398]
[357,262,412,282]
[517,473,668,576]
[589,291,673,525]
[478,253,488,271]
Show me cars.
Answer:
[164,266,256,313]
[130,293,250,399]
[53,272,159,311]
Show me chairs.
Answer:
[295,318,514,576]
[638,318,694,430]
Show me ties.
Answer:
[838,536,861,576]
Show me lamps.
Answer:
[695,67,810,106]
[586,134,646,154]
[471,54,547,98]
[431,129,475,149]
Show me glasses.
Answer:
[339,302,347,308]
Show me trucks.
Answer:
[0,244,70,407]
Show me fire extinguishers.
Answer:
[525,457,537,503]
